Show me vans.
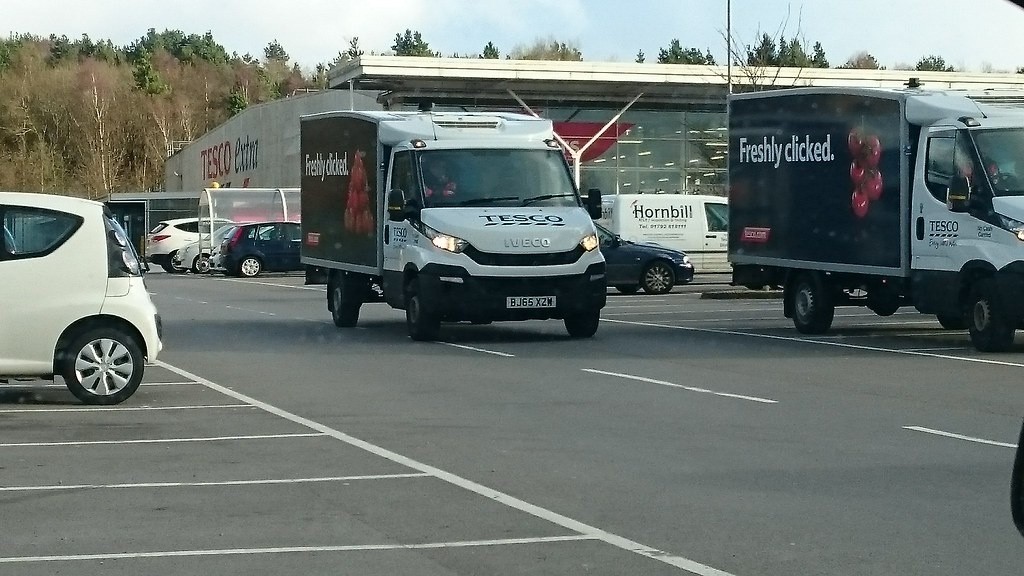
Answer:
[594,193,732,274]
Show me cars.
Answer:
[594,221,694,295]
[219,221,307,277]
[209,244,232,276]
[178,223,236,273]
[145,216,235,274]
[0,192,164,406]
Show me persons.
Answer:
[424,157,458,198]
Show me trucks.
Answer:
[297,110,607,342]
[724,87,1024,354]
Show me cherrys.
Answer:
[848,127,882,216]
[343,151,378,239]
